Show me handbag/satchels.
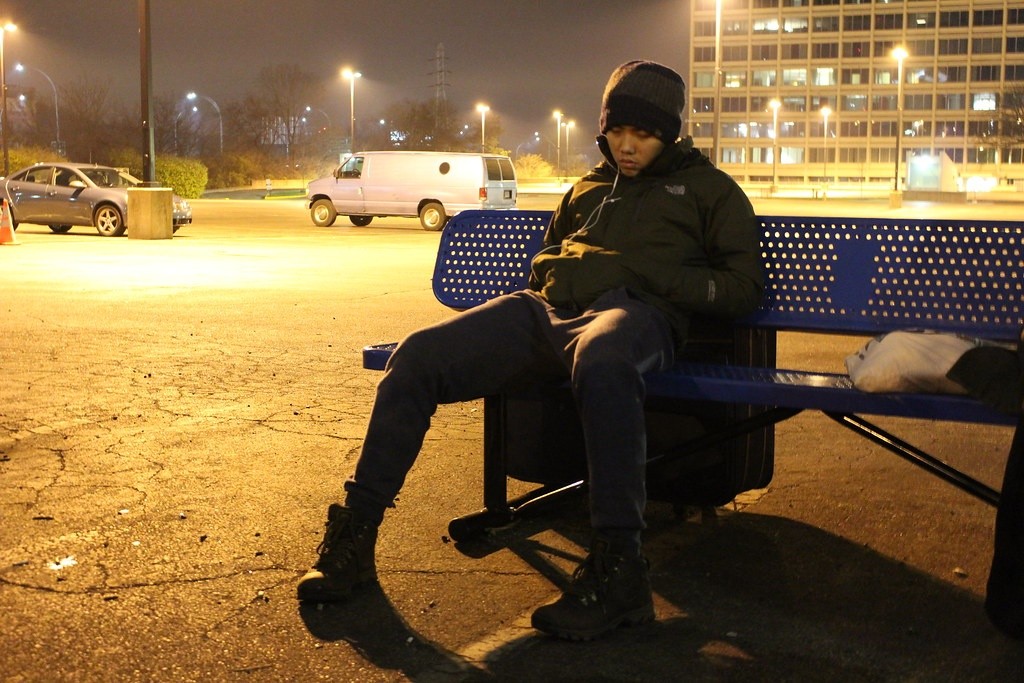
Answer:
[844,331,982,394]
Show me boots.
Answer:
[531,529,656,642]
[295,505,380,601]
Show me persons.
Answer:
[295,59,766,643]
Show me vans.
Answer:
[307,150,519,231]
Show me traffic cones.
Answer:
[0,199,21,245]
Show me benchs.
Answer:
[362,209,1024,616]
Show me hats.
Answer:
[601,59,686,142]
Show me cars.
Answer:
[0,162,192,236]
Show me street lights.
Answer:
[306,106,331,128]
[892,48,909,190]
[188,93,222,153]
[17,65,60,156]
[535,132,550,158]
[343,71,361,153]
[819,107,832,183]
[516,137,539,161]
[769,100,781,185]
[562,121,575,176]
[554,112,561,180]
[477,105,490,153]
[0,23,18,178]
[174,107,197,156]
